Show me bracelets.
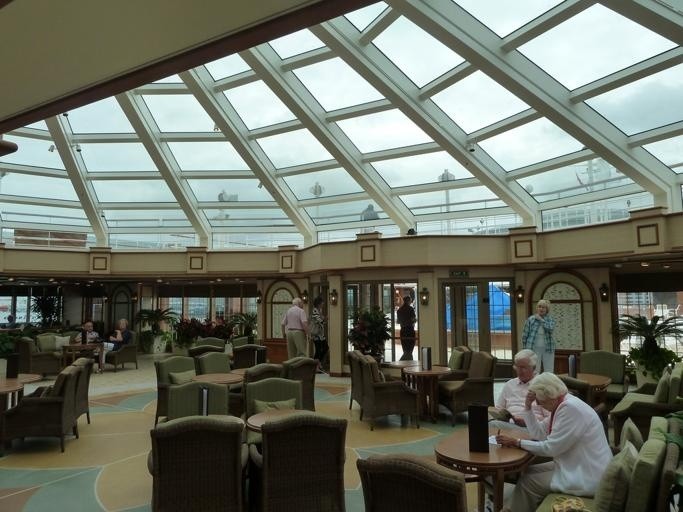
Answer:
[517,438,520,448]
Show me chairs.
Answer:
[438,351,497,427]
[347,349,391,421]
[607,363,683,446]
[356,452,468,512]
[556,376,608,444]
[647,415,682,448]
[250,411,347,510]
[359,354,421,431]
[154,334,320,445]
[616,303,682,326]
[0,322,138,454]
[447,345,473,381]
[146,415,248,511]
[580,351,629,399]
[535,440,680,511]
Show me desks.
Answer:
[390,359,439,380]
[555,373,612,406]
[433,427,535,511]
[402,366,452,423]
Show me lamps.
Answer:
[418,286,429,307]
[598,283,608,302]
[467,401,490,454]
[567,353,577,379]
[514,285,524,304]
[327,288,337,306]
[299,289,308,304]
[421,346,432,370]
[254,289,263,304]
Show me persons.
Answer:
[485,349,551,511]
[310,297,331,376]
[75,322,99,347]
[280,298,310,361]
[519,299,555,372]
[395,296,416,361]
[494,372,612,511]
[96,318,130,372]
[5,316,15,327]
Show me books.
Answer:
[486,403,516,423]
[497,428,529,449]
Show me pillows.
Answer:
[591,446,635,511]
[449,348,464,371]
[377,369,385,383]
[653,371,670,404]
[623,440,639,460]
[619,417,644,452]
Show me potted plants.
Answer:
[607,311,683,388]
[131,306,180,356]
